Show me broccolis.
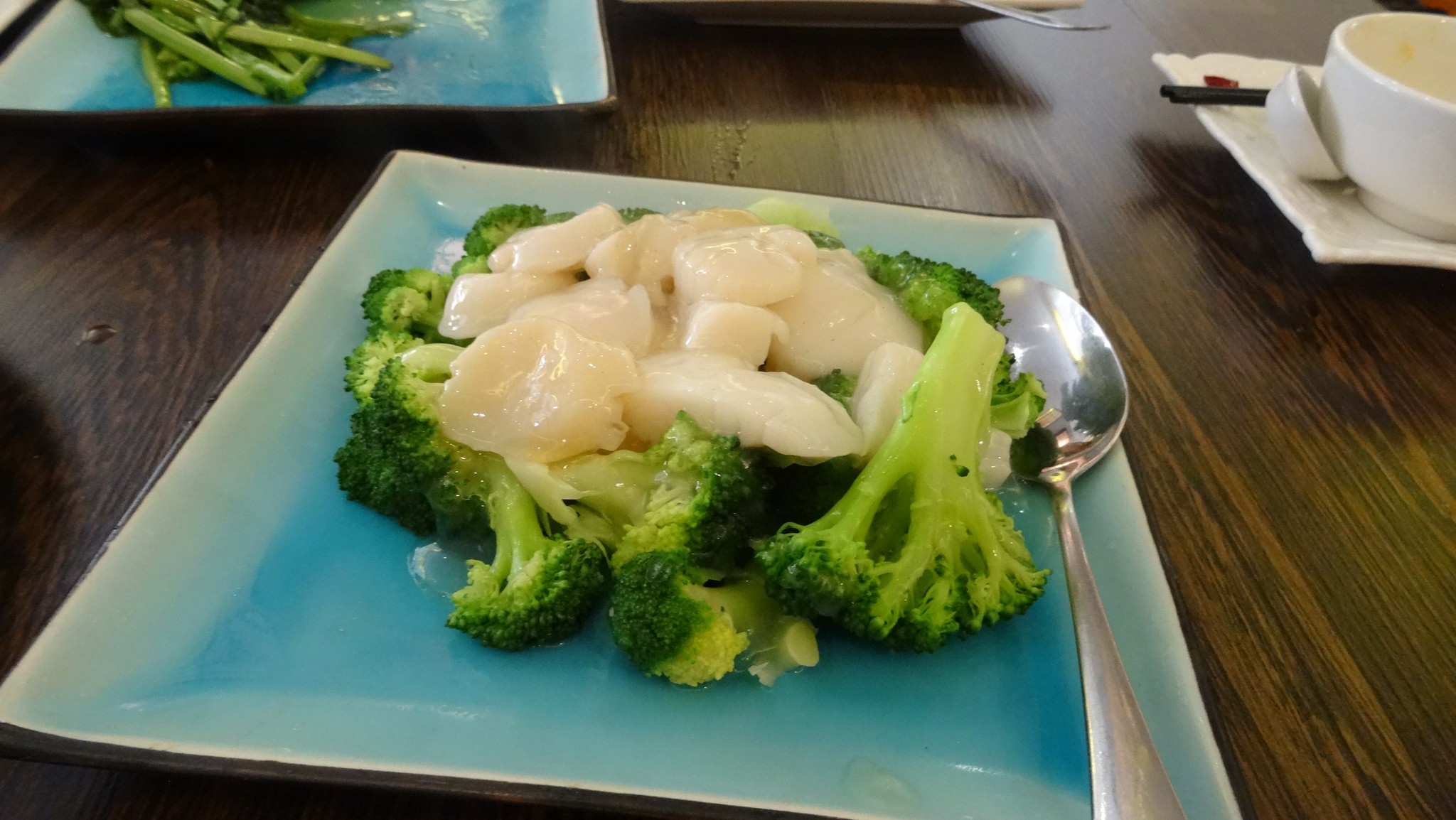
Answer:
[333,198,1053,688]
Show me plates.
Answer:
[605,0,1083,29]
[0,147,1243,819]
[2,0,617,115]
[1151,52,1455,270]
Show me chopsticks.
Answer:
[1159,84,1268,105]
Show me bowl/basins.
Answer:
[1264,64,1343,182]
[1318,12,1456,243]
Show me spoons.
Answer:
[989,273,1186,820]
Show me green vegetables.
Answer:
[83,0,412,108]
